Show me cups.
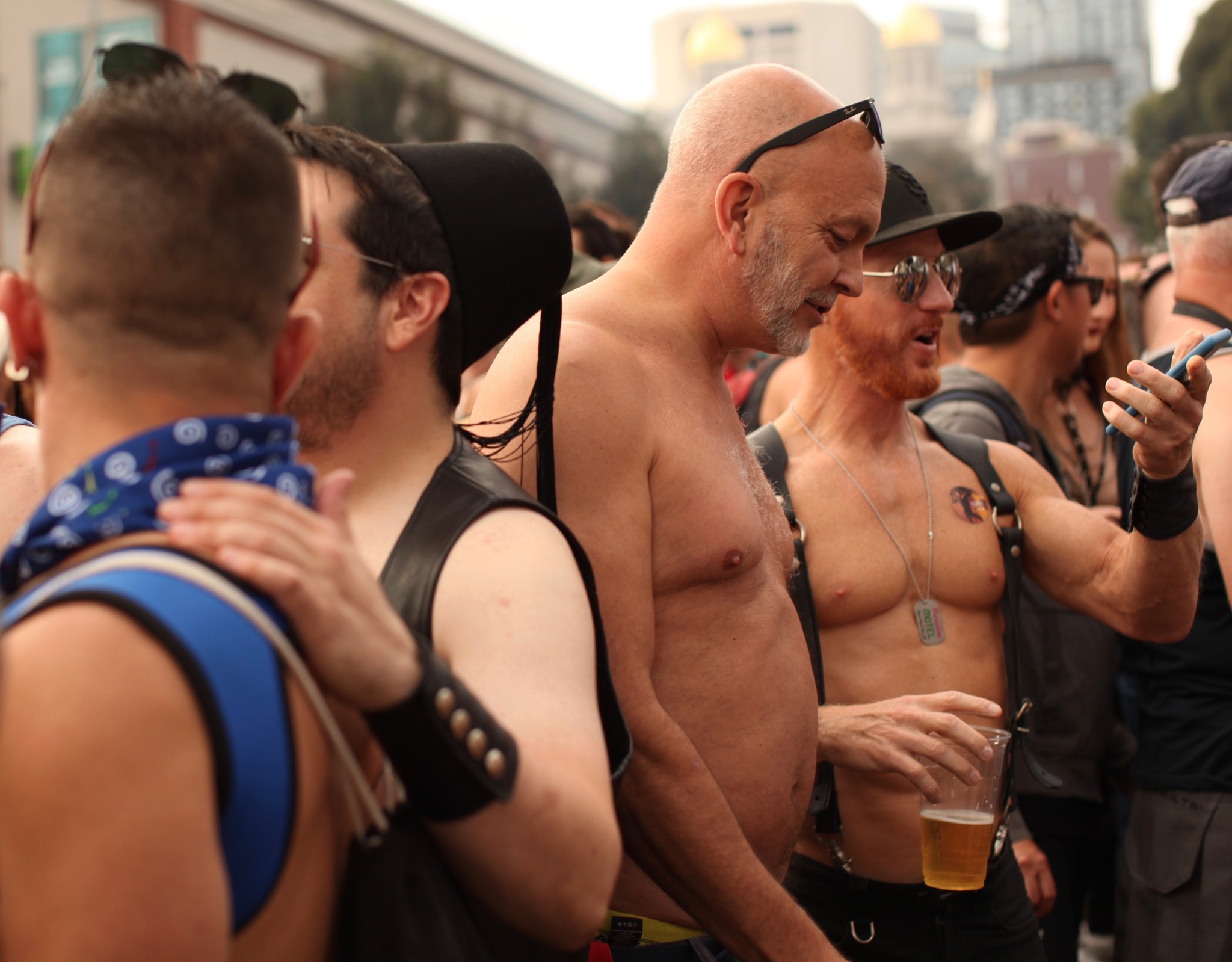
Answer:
[921,727,1011,891]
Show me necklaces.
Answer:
[793,405,946,644]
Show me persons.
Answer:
[0,124,1232,962]
[159,115,632,962]
[471,63,889,961]
[745,158,1204,962]
[0,42,389,962]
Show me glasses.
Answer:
[732,98,885,174]
[1066,276,1104,306]
[96,41,307,124]
[863,252,964,305]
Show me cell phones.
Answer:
[1105,327,1232,437]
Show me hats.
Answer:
[1159,139,1232,227]
[381,142,573,515]
[864,160,1003,252]
